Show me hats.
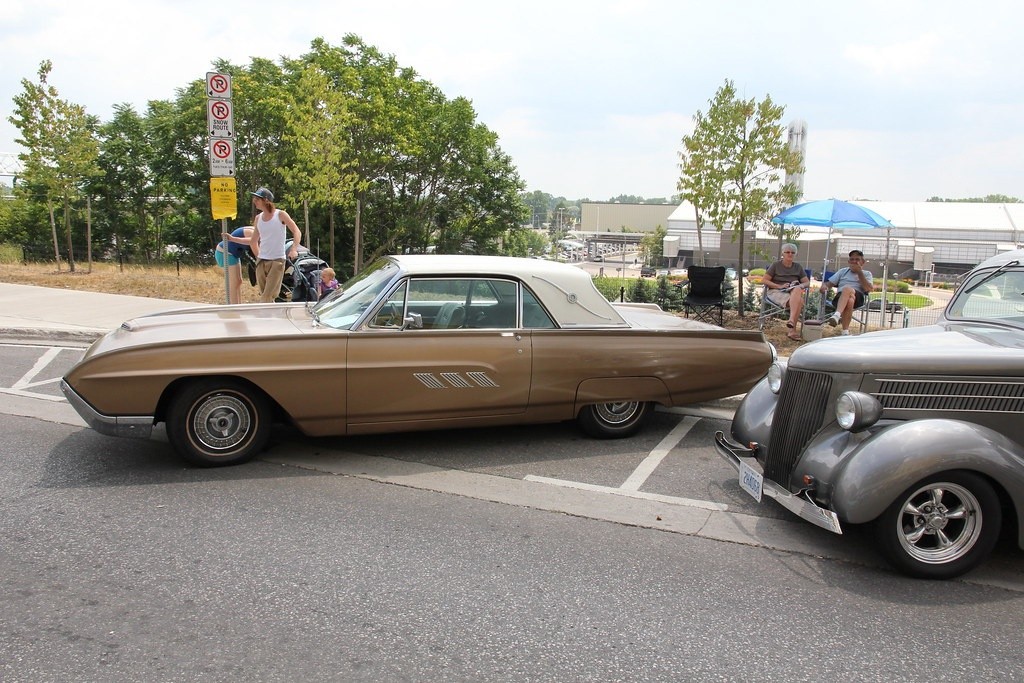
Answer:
[250,188,274,201]
[849,250,863,257]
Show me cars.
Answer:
[713,247,1024,579]
[593,255,605,262]
[640,266,750,281]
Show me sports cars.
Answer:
[59,254,778,465]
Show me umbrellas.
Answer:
[771,197,896,321]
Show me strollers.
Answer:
[243,238,343,301]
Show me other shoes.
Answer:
[788,333,801,341]
[829,315,838,328]
[842,330,850,336]
[786,321,794,328]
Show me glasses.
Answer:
[849,258,860,261]
[784,251,795,254]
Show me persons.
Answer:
[819,250,873,336]
[214,226,261,304]
[223,187,302,303]
[761,243,809,341]
[316,267,338,302]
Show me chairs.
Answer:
[674,267,733,327]
[430,303,465,330]
[821,272,870,333]
[759,266,812,339]
